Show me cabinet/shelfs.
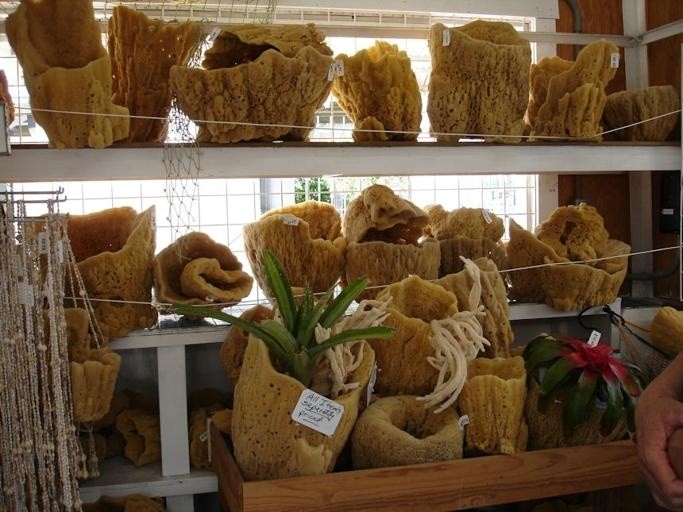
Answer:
[1,0,682,512]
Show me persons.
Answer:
[632,347,682,512]
